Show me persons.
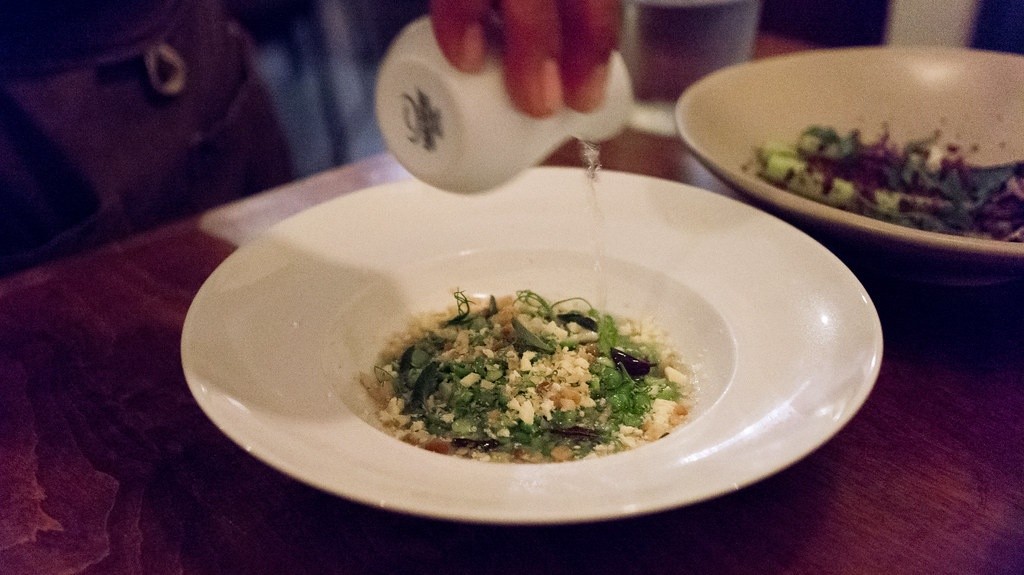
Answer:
[0,1,620,264]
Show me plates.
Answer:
[179,164,885,525]
[672,42,1024,294]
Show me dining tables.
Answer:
[0,126,1024,575]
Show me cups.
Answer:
[376,10,634,199]
